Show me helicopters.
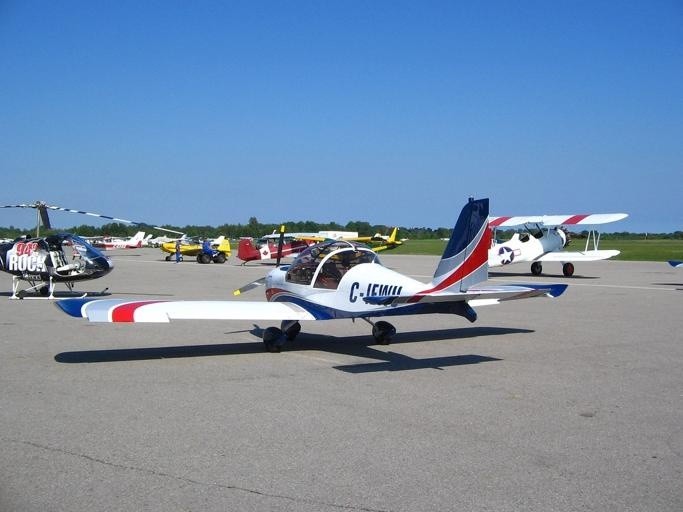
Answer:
[0,199,187,300]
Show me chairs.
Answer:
[49,249,80,272]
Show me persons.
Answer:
[174,239,183,263]
